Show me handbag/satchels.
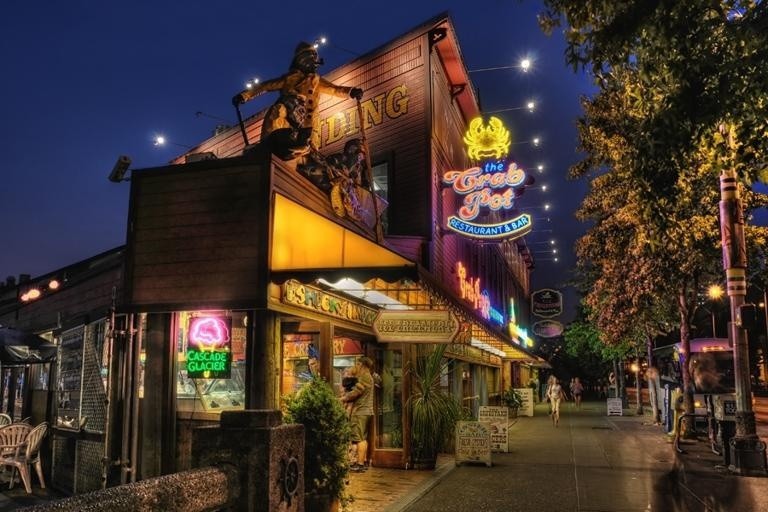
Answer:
[547,395,551,402]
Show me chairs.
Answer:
[0,413,47,494]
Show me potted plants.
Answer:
[279,367,356,512]
[504,386,523,419]
[403,343,457,471]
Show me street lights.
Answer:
[707,284,723,339]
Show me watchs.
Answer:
[570,378,575,401]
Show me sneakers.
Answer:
[350,461,368,471]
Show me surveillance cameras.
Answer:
[108,154,131,184]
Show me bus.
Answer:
[646,338,756,423]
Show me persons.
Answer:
[309,356,342,383]
[341,356,374,471]
[548,374,566,426]
[572,377,584,410]
[340,367,359,418]
[234,41,363,163]
